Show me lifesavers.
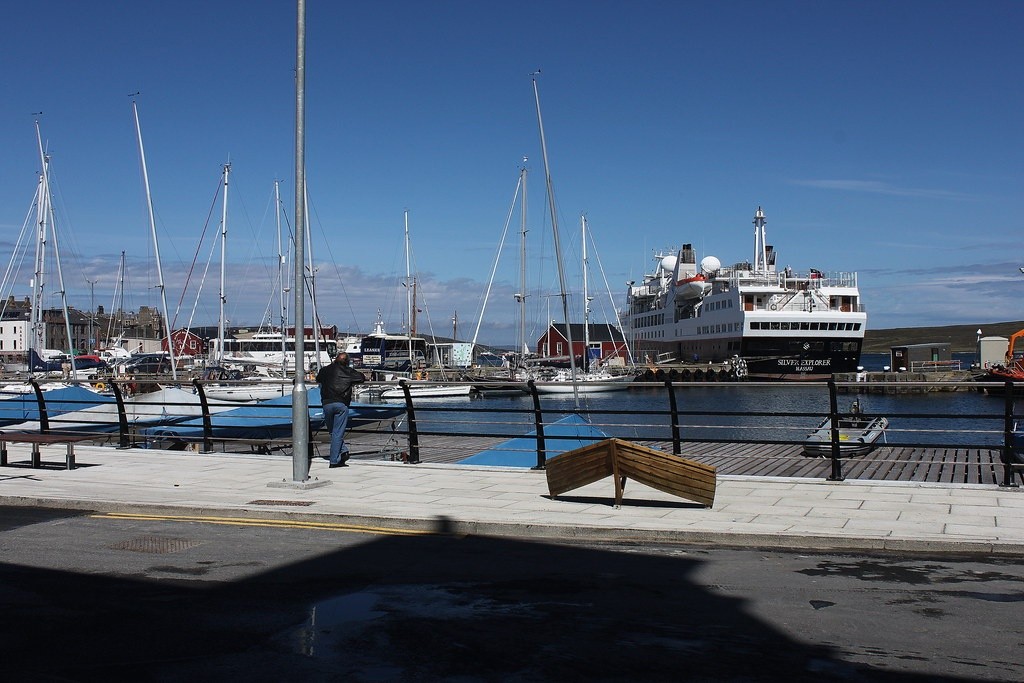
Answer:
[96,382,104,389]
[771,304,778,311]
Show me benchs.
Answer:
[0,433,89,470]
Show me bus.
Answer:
[208,338,340,375]
[344,333,428,371]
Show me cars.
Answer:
[106,353,177,374]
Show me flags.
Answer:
[811,269,821,278]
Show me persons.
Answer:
[992,363,1004,370]
[502,354,512,367]
[316,352,364,468]
[201,356,205,372]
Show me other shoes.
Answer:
[328,451,349,467]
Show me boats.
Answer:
[990,360,1024,381]
[801,395,891,460]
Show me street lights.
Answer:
[84,277,100,352]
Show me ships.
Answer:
[622,200,869,377]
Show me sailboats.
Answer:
[0,90,413,444]
[376,167,643,400]
